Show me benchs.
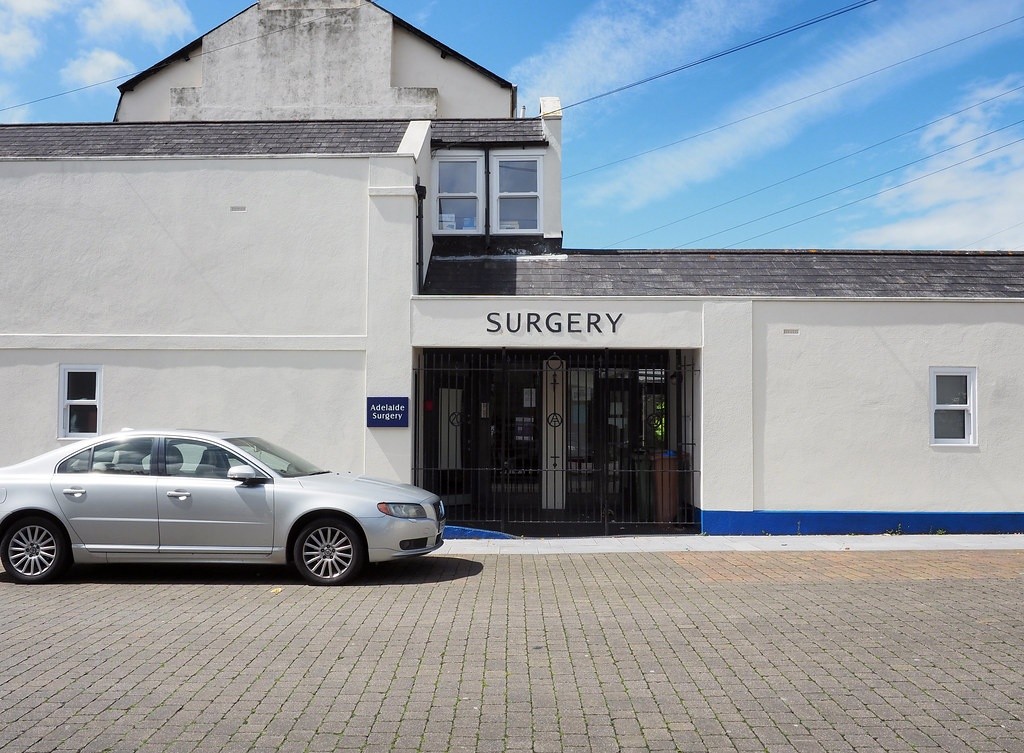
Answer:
[70,448,139,474]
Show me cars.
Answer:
[0,426,443,585]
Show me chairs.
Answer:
[165,444,188,477]
[194,449,226,478]
